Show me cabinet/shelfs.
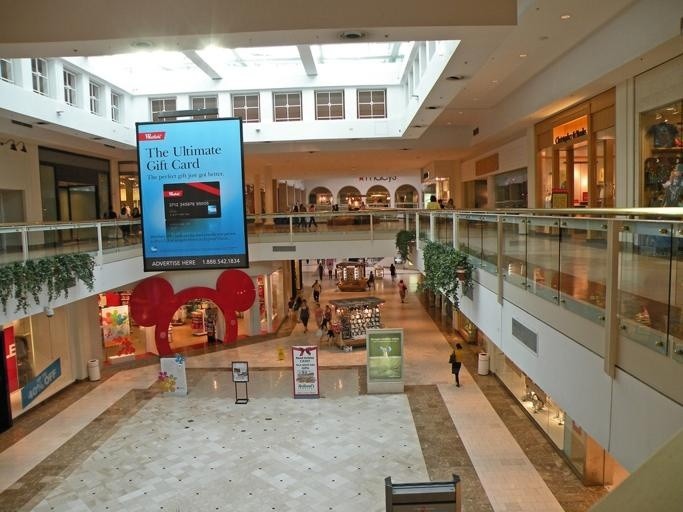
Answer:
[190,308,208,337]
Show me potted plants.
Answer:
[0,253,97,318]
[395,228,426,264]
[417,239,474,316]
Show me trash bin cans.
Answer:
[87,359,101,381]
[518,213,529,235]
[478,353,490,376]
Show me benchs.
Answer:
[108,352,137,364]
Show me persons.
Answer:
[291,205,299,228]
[288,296,332,338]
[318,262,323,280]
[104,204,118,246]
[308,204,318,229]
[327,263,333,280]
[117,207,130,245]
[437,200,447,209]
[298,203,309,229]
[366,270,374,289]
[131,207,141,244]
[311,279,321,307]
[389,263,395,282]
[396,279,407,303]
[451,342,461,388]
[445,198,455,210]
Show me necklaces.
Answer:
[425,196,441,209]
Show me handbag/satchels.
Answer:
[449,350,456,363]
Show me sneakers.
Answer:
[456,384,460,388]
